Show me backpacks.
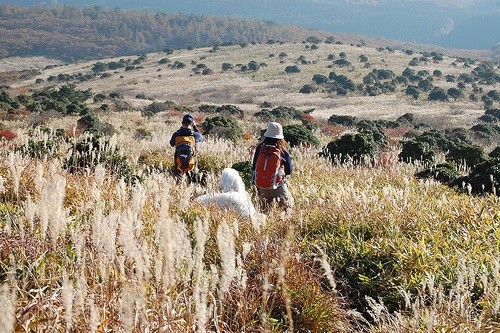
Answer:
[174,130,195,174]
[251,141,286,190]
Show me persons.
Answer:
[170,114,203,181]
[251,121,295,209]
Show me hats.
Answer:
[264,122,284,140]
